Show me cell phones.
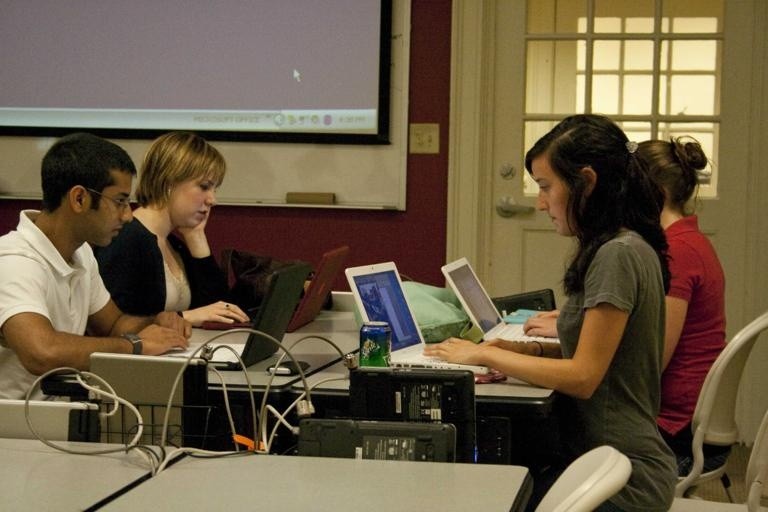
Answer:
[267,359,308,376]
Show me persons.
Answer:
[91,132,250,328]
[524,135,732,478]
[0,134,192,402]
[422,115,678,510]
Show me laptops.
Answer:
[345,260,489,375]
[202,245,350,333]
[159,262,311,371]
[441,256,560,345]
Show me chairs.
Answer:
[534,446,633,511]
[676,311,767,503]
[664,412,768,512]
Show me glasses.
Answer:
[82,185,131,215]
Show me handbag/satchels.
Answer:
[231,249,331,312]
[354,281,470,343]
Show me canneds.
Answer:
[359,321,391,367]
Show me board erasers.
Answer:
[286,192,335,205]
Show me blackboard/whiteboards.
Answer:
[0,0,411,211]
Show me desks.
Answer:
[38,312,359,397]
[293,336,555,465]
[0,438,177,512]
[93,451,536,511]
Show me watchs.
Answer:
[121,333,143,355]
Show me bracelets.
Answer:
[533,341,543,356]
[176,310,183,318]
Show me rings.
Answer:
[225,304,229,308]
